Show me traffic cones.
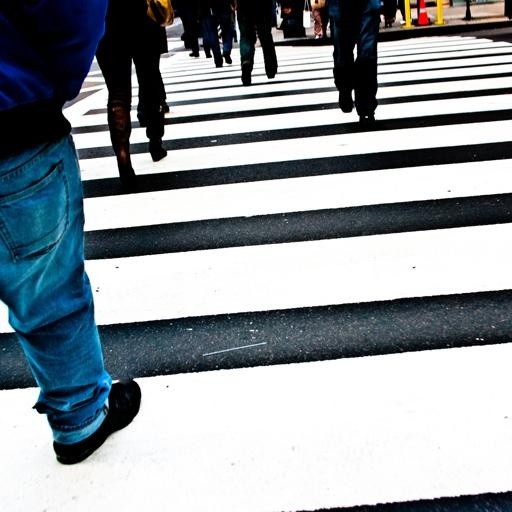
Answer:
[418,0,430,26]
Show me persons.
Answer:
[323,0,385,125]
[382,0,405,28]
[136,26,169,126]
[178,1,307,85]
[319,0,329,39]
[95,0,171,182]
[0,0,142,465]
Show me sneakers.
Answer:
[215,61,222,67]
[223,51,232,64]
[241,59,253,86]
[47,380,141,464]
[267,74,275,77]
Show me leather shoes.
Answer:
[361,116,376,130]
[339,77,354,112]
[190,51,199,57]
[206,54,212,58]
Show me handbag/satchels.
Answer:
[303,9,314,28]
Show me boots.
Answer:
[107,102,135,183]
[146,99,169,162]
